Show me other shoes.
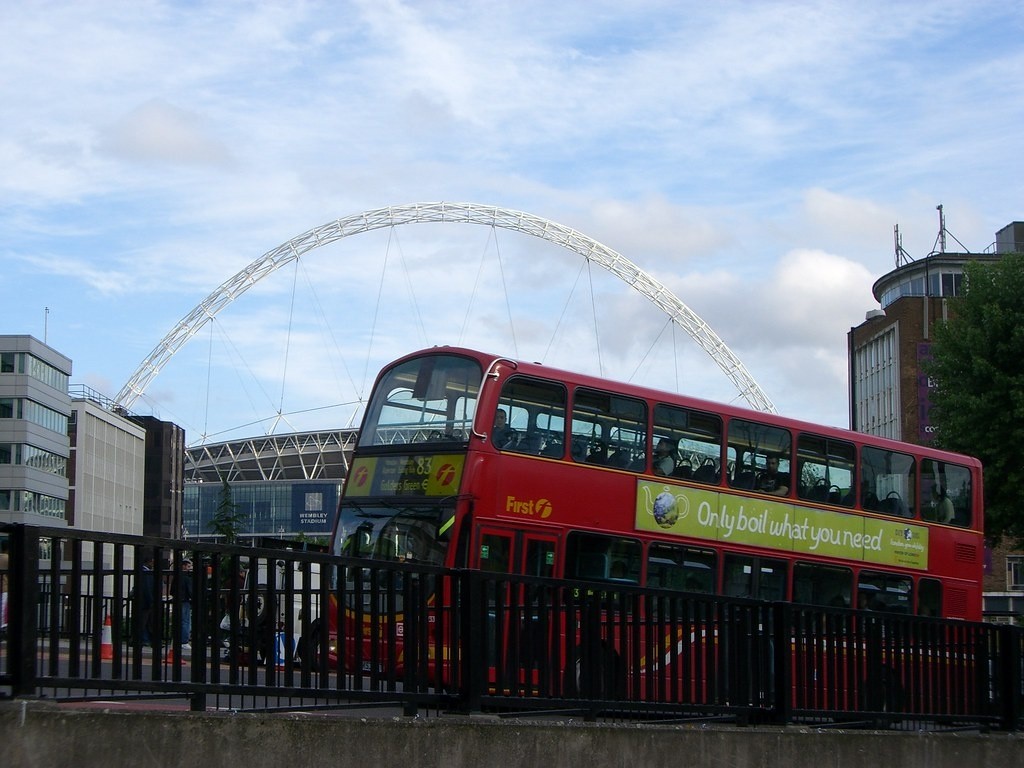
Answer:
[182,643,192,650]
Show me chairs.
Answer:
[428,426,972,527]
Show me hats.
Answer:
[239,567,248,572]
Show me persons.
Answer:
[609,560,932,623]
[651,437,675,477]
[931,483,956,523]
[492,408,516,448]
[170,560,193,651]
[755,453,791,496]
[130,555,154,648]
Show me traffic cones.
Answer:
[164,637,186,665]
[99,615,116,660]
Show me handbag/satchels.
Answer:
[220,613,231,631]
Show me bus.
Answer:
[316,343,986,713]
